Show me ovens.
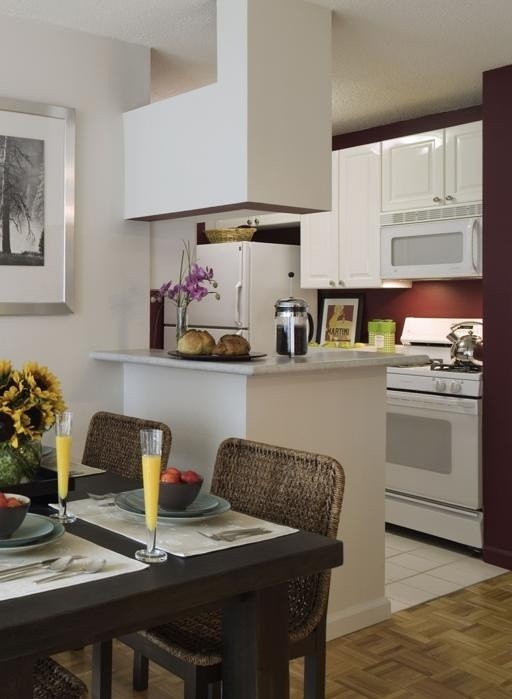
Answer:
[385,389,484,559]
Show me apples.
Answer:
[1,492,22,511]
[162,468,200,483]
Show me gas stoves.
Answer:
[387,344,484,398]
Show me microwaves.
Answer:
[379,200,483,280]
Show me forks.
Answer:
[195,528,274,543]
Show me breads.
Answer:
[213,334,249,355]
[176,328,215,355]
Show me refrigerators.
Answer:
[182,241,318,355]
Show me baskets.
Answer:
[204,228,257,243]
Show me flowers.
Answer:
[0,359,67,484]
[157,254,218,333]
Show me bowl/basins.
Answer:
[1,490,31,541]
[148,477,206,511]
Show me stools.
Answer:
[14,651,91,697]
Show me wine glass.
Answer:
[136,428,169,564]
[50,412,77,526]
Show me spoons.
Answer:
[88,490,113,501]
[1,548,107,584]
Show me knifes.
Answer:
[97,502,114,509]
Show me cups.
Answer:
[367,318,397,356]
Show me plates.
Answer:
[1,512,66,555]
[166,348,267,361]
[113,489,232,528]
[40,444,58,467]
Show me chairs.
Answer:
[125,436,342,699]
[80,410,174,697]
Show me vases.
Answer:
[0,439,44,495]
[176,307,189,341]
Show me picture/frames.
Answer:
[0,97,81,316]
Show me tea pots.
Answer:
[445,320,483,366]
[272,297,314,358]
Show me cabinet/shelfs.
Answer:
[299,142,412,289]
[382,121,484,219]
